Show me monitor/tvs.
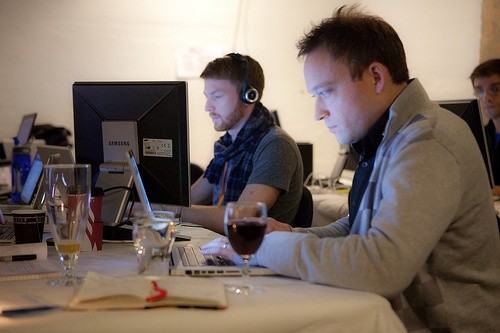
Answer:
[434,100,496,190]
[71,80,190,242]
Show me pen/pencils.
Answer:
[0,254,37,262]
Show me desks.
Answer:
[309,176,354,228]
[0,211,408,333]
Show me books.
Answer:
[67,272,226,309]
[0,259,60,282]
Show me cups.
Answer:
[11,210,46,260]
[134,209,175,277]
[68,185,104,253]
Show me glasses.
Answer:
[473,86,500,97]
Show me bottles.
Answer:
[12,146,31,203]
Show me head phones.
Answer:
[227,53,259,104]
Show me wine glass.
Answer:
[223,201,266,294]
[45,163,91,288]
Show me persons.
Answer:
[198,2,500,333]
[460,58,500,195]
[123,52,303,238]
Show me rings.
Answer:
[224,244,226,248]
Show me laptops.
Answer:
[14,113,74,205]
[125,149,282,277]
